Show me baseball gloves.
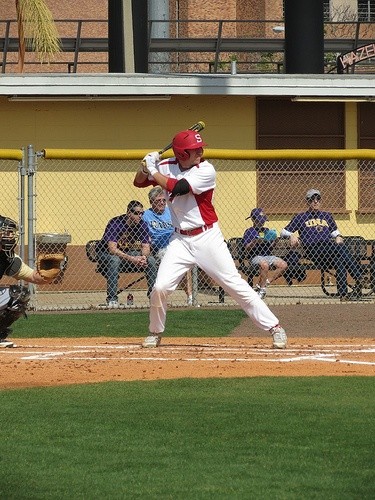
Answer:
[36,253,64,281]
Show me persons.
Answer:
[0,216,64,348]
[280,189,368,301]
[133,130,287,349]
[141,187,174,266]
[97,200,157,306]
[243,209,287,299]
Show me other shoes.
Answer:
[108,301,118,306]
[259,289,266,299]
[256,283,266,290]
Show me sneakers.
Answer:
[0,339,13,348]
[270,327,288,348]
[142,333,161,347]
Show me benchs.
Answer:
[86,237,375,302]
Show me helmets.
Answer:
[0,215,16,252]
[171,130,208,161]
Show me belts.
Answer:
[175,223,213,236]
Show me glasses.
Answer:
[308,195,321,200]
[130,208,145,215]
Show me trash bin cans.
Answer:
[35,233,72,284]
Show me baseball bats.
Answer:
[142,121,206,167]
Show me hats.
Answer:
[251,208,268,223]
[305,189,321,197]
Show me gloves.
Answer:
[140,152,160,175]
[144,153,158,176]
